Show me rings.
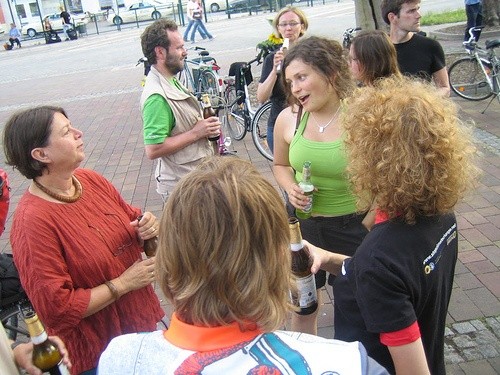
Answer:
[152,227,155,231]
[151,271,154,277]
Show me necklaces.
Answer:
[32,176,82,202]
[310,107,341,133]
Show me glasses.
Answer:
[277,22,301,27]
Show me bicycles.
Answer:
[447,24,500,114]
[135,47,274,163]
[341,28,361,47]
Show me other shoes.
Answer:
[191,40,198,44]
[184,39,190,42]
[210,34,217,41]
[203,38,209,41]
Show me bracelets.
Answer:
[11,342,26,349]
[105,281,119,300]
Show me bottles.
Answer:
[25,311,69,375]
[277,37,290,75]
[137,214,159,258]
[202,94,221,143]
[486,69,493,82]
[289,217,318,315]
[295,160,315,220]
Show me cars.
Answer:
[106,0,306,25]
[21,12,88,37]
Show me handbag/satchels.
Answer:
[193,12,202,20]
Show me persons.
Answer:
[0,0,486,375]
